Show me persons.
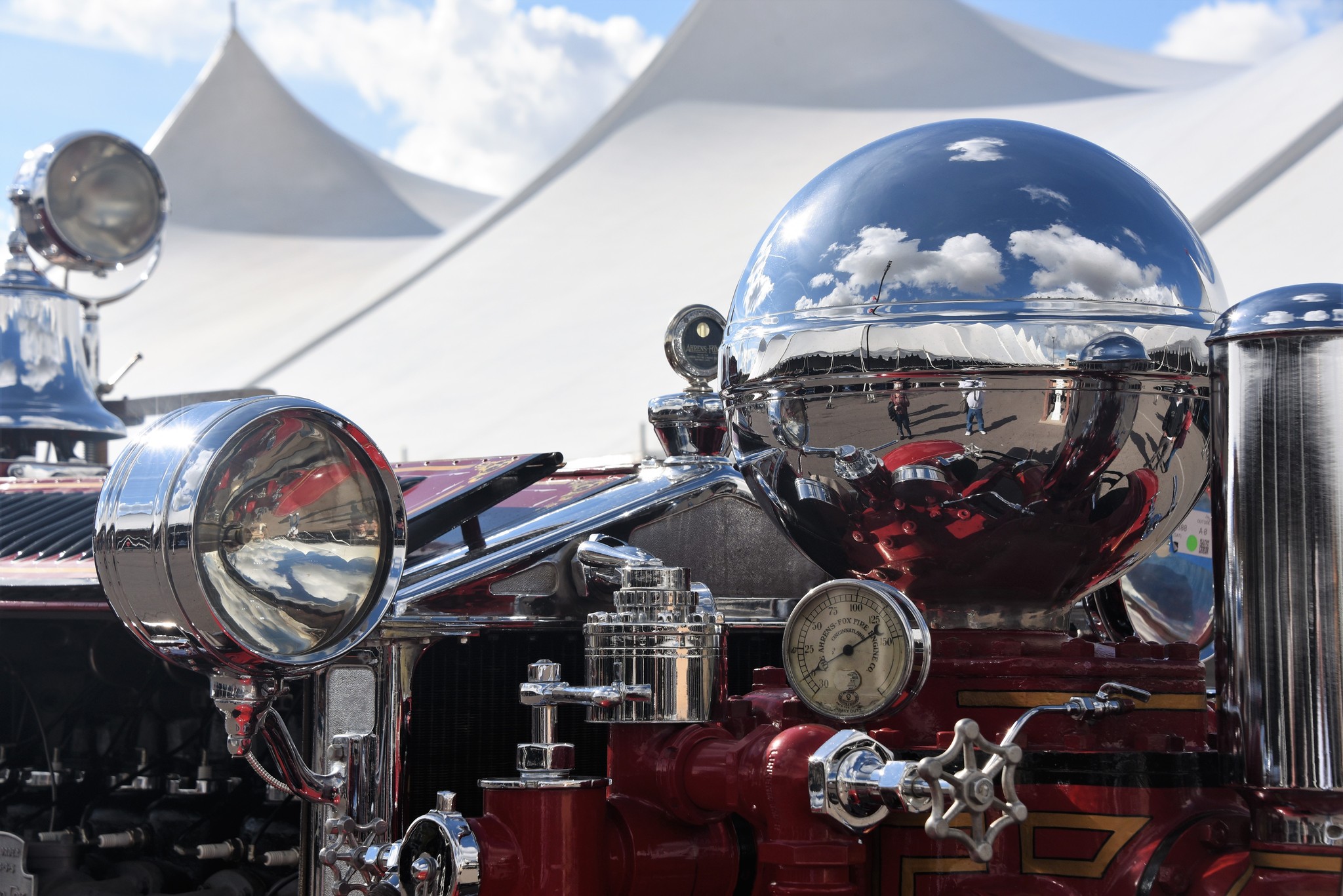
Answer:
[891,387,911,440]
[958,379,986,435]
[892,381,903,389]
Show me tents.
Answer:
[100,0,505,400]
[244,0,1343,494]
[747,322,1053,365]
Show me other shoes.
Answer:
[1144,463,1149,468]
[900,436,904,440]
[908,436,912,439]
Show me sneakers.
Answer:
[979,431,987,434]
[965,432,971,436]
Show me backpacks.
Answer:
[887,393,897,422]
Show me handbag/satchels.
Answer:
[959,397,968,414]
